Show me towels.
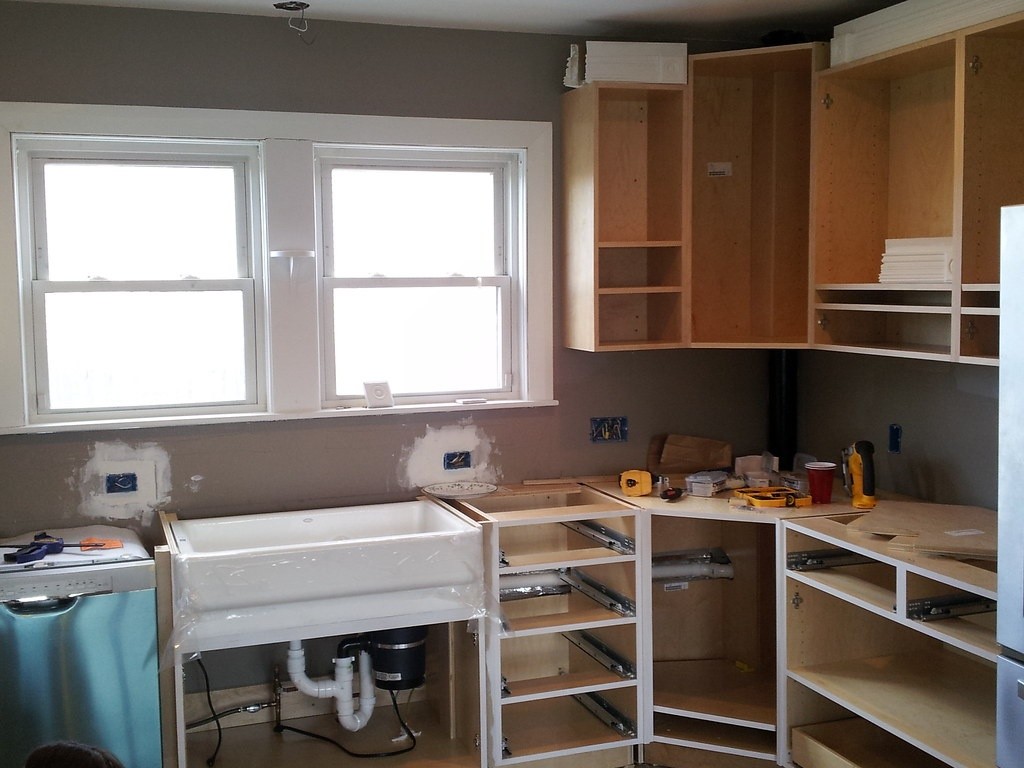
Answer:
[878,237,955,283]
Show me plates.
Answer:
[424,482,497,499]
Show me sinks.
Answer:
[171,495,486,655]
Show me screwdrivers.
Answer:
[660,486,687,499]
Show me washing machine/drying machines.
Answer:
[0,526,163,768]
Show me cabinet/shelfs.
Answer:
[556,10,1024,367]
[780,513,998,768]
[427,477,643,768]
[643,509,781,761]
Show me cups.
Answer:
[805,461,837,504]
